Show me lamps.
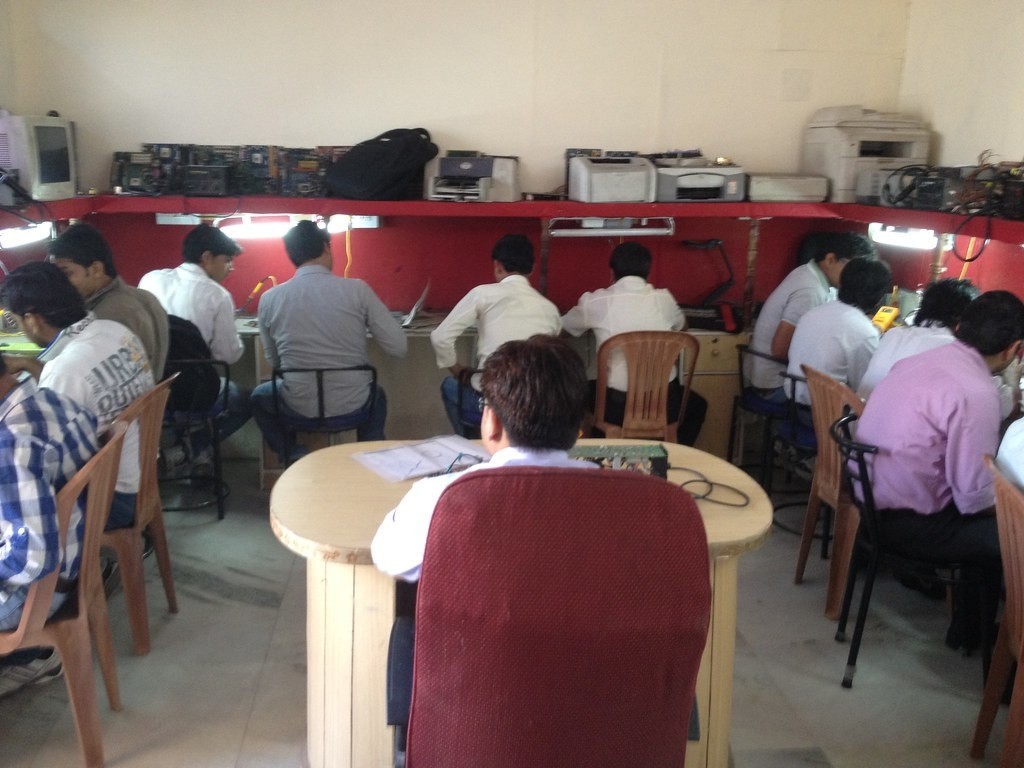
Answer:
[546,217,676,238]
[867,221,955,253]
[213,212,344,238]
[0,222,52,249]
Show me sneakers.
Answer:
[0,647,63,696]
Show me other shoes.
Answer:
[101,557,121,592]
[886,554,943,597]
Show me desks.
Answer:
[235,312,758,454]
[270,436,775,768]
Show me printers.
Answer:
[651,156,745,203]
[428,150,522,203]
[803,107,931,203]
[569,156,657,203]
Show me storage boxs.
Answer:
[745,171,828,203]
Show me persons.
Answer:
[0,261,156,600]
[371,333,600,582]
[847,290,1024,649]
[560,242,709,446]
[0,351,104,698]
[784,255,893,426]
[137,223,252,477]
[752,232,876,412]
[50,222,169,384]
[430,234,563,437]
[250,220,408,469]
[848,278,972,440]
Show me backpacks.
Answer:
[322,128,438,199]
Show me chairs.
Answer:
[385,468,712,767]
[726,343,1024,768]
[1,318,230,768]
[457,366,485,438]
[272,363,378,471]
[584,331,701,442]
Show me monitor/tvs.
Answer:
[22,115,76,201]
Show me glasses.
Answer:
[476,398,495,413]
